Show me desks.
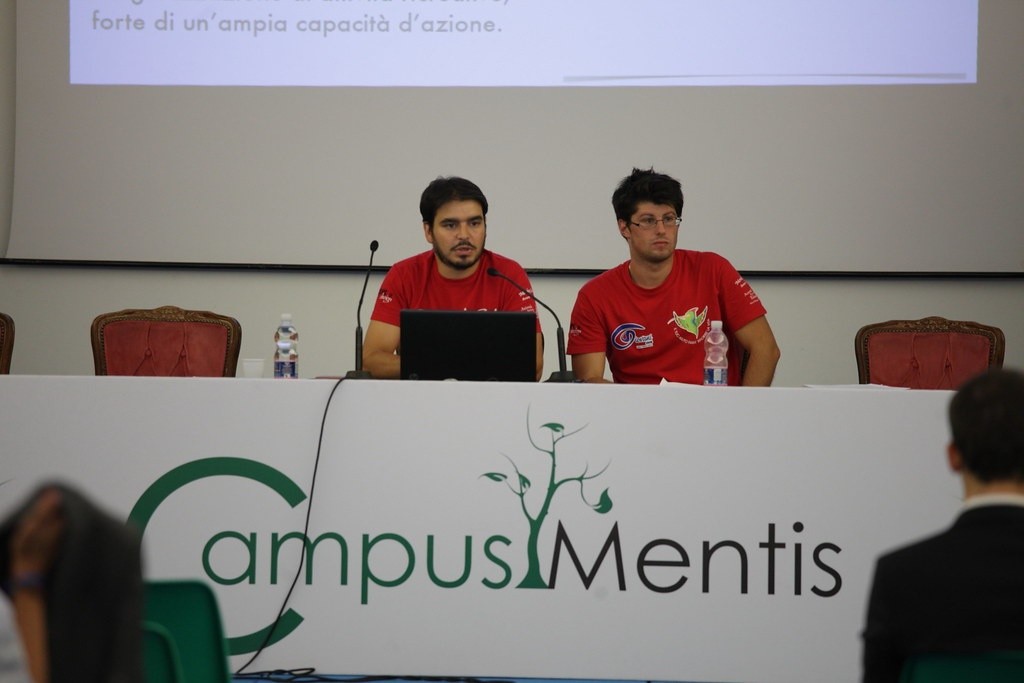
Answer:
[0,373,963,683]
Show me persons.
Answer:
[566,171,780,387]
[860,370,1024,683]
[363,178,544,381]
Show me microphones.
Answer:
[487,266,577,383]
[345,240,379,379]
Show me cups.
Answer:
[243,359,265,377]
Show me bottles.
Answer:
[273,314,298,379]
[703,321,728,386]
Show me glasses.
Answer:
[625,217,684,230]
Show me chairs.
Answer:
[90,306,241,379]
[852,316,1007,390]
[137,579,234,683]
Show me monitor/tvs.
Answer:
[400,309,537,382]
